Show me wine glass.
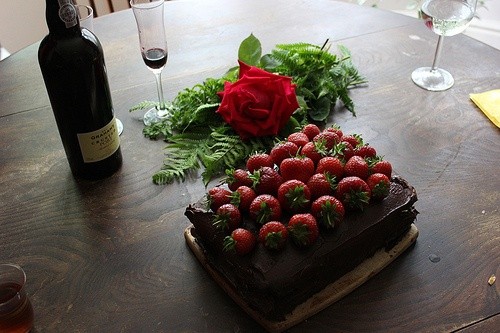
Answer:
[130,0,183,129]
[410,0,477,92]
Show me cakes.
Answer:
[184,124,421,322]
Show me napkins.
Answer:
[470,86,500,129]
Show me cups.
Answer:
[74,5,94,33]
[0,264,32,333]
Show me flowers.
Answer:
[122,36,358,187]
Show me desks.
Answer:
[0,0,500,333]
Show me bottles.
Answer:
[38,0,123,184]
